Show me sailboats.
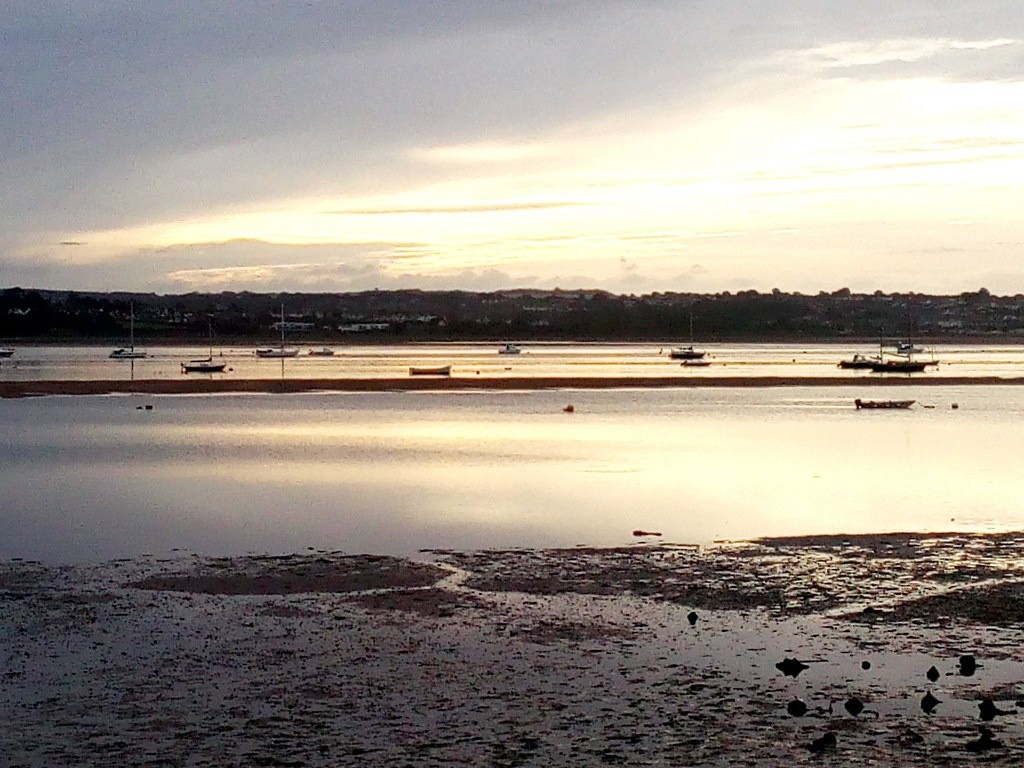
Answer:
[108,302,147,359]
[869,297,926,372]
[256,302,300,358]
[670,313,707,358]
[179,314,227,372]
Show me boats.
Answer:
[856,398,917,409]
[499,343,521,355]
[837,355,880,369]
[409,364,452,374]
[310,347,333,356]
[897,339,924,353]
[1,349,15,358]
[916,342,940,366]
[680,360,711,366]
